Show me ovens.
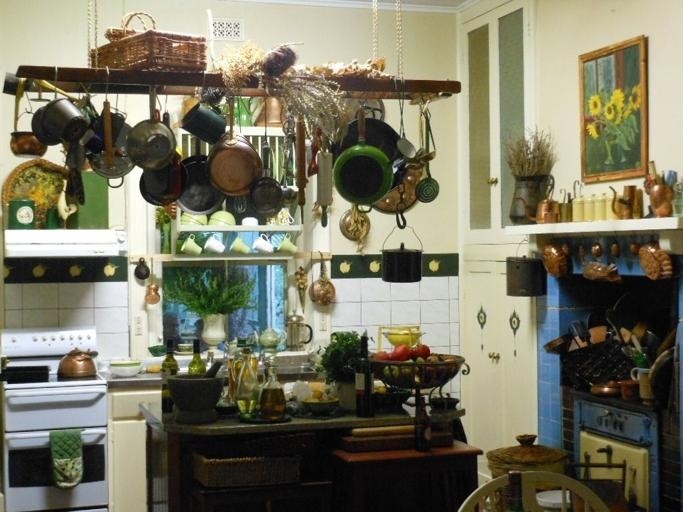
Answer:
[3,386,109,511]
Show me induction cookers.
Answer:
[2,358,108,389]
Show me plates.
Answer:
[173,350,194,355]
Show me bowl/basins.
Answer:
[303,398,340,414]
[10,131,48,157]
[177,343,193,351]
[127,120,176,169]
[209,210,236,225]
[617,380,639,401]
[207,138,262,197]
[242,216,258,226]
[41,98,83,137]
[109,361,144,377]
[379,326,420,336]
[383,331,426,345]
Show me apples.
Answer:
[376,344,455,383]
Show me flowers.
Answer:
[587,85,642,163]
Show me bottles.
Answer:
[585,194,596,222]
[237,351,257,420]
[258,356,287,420]
[605,195,619,220]
[596,193,607,221]
[8,199,35,229]
[415,397,433,452]
[572,194,584,221]
[227,338,258,399]
[188,339,206,375]
[354,336,374,417]
[162,340,179,421]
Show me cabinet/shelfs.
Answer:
[459,245,538,482]
[335,440,483,512]
[183,477,331,511]
[458,0,537,245]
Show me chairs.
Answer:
[456,471,611,512]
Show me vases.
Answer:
[510,175,555,225]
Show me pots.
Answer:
[333,107,401,160]
[139,112,189,205]
[506,257,547,297]
[176,139,225,213]
[180,104,226,144]
[379,243,423,282]
[33,106,61,145]
[253,146,282,217]
[2,366,50,383]
[89,123,135,189]
[335,109,393,211]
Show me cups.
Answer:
[229,236,251,255]
[180,234,202,256]
[44,208,60,228]
[631,367,654,380]
[252,233,274,254]
[204,234,225,254]
[277,233,297,254]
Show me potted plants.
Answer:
[163,267,257,345]
[313,332,364,411]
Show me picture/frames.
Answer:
[579,35,648,184]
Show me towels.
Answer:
[50,431,83,488]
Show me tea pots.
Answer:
[517,196,561,223]
[57,347,99,376]
[253,328,287,352]
[284,310,312,352]
